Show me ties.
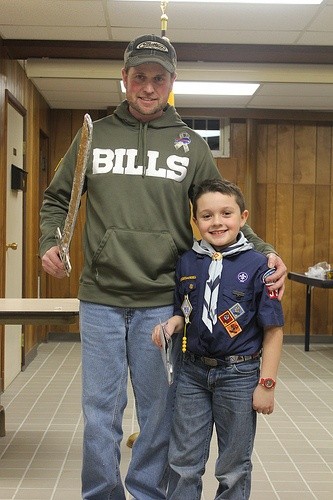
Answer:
[191,240,254,333]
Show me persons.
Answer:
[34,33,288,500]
[151,178,287,500]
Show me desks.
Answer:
[287,271,333,351]
[0,297,81,439]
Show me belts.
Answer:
[185,350,260,367]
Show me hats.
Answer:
[123,34,177,76]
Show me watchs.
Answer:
[258,376,276,389]
